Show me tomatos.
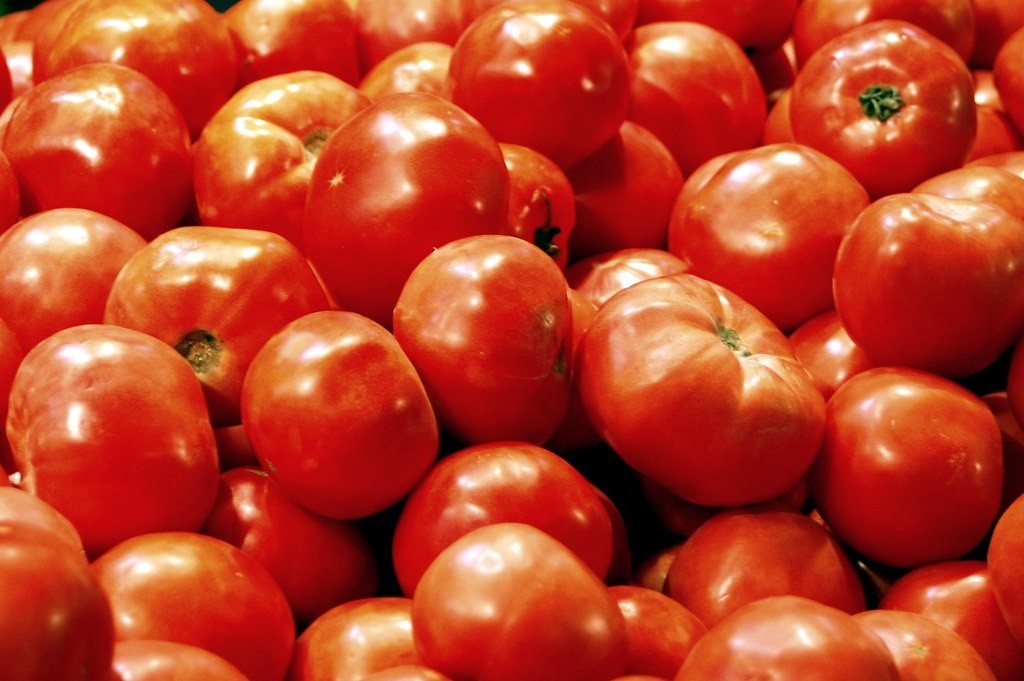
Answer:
[0,1,1024,681]
